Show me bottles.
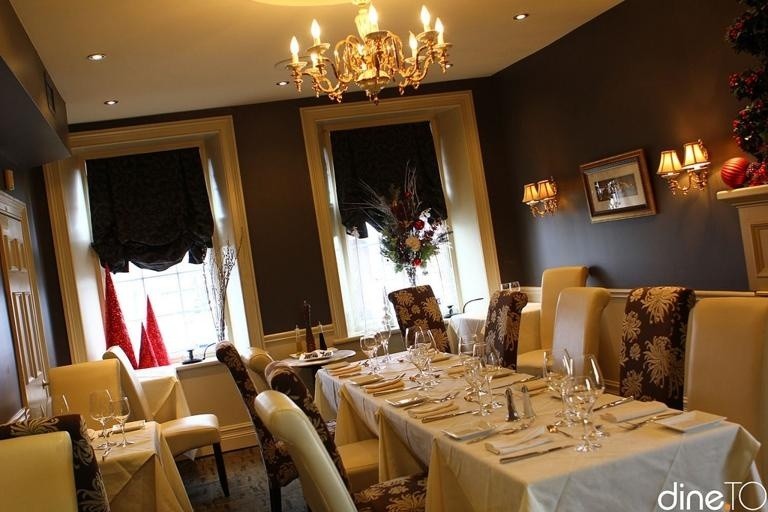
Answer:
[519,383,536,419]
[295,327,302,355]
[318,325,328,354]
[503,388,521,422]
[303,304,316,352]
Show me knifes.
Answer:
[593,394,634,412]
[421,409,474,423]
[499,444,574,465]
[101,447,112,457]
[373,385,421,397]
[339,372,362,378]
[494,375,542,389]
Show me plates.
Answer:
[112,419,145,434]
[288,347,338,361]
[348,372,385,385]
[492,369,516,378]
[322,361,350,371]
[442,420,493,440]
[384,393,426,406]
[655,408,727,432]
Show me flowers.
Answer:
[349,150,455,287]
[721,1,767,163]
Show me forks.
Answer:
[368,371,406,385]
[617,412,685,429]
[403,391,460,411]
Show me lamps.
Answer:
[653,138,710,200]
[283,0,453,101]
[520,170,563,219]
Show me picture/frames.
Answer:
[575,149,658,222]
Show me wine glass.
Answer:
[88,389,117,447]
[108,396,135,447]
[540,348,609,453]
[359,323,393,375]
[500,281,521,291]
[457,334,504,415]
[403,325,442,392]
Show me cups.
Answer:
[25,394,70,420]
